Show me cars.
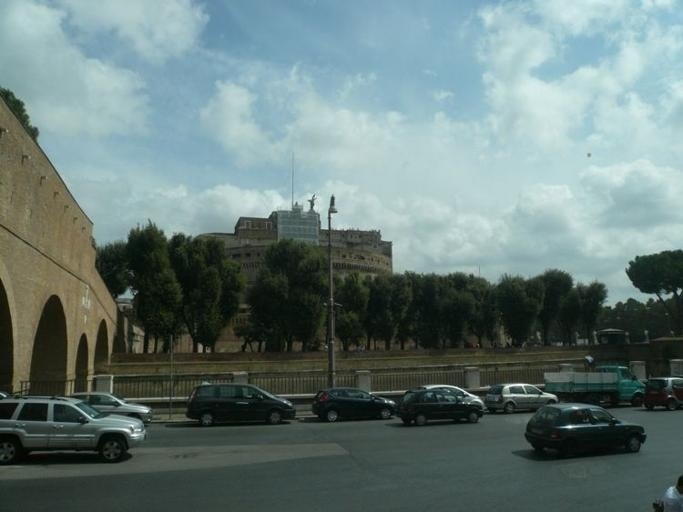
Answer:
[399,384,485,426]
[72,391,153,427]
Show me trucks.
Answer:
[542,363,646,407]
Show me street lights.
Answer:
[327,193,339,386]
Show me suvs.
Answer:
[0,395,146,466]
[485,382,559,415]
[311,387,397,423]
[524,403,648,457]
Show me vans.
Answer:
[644,376,683,411]
[185,382,297,425]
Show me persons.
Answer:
[658,475,683,512]
[489,340,528,348]
[357,344,363,353]
